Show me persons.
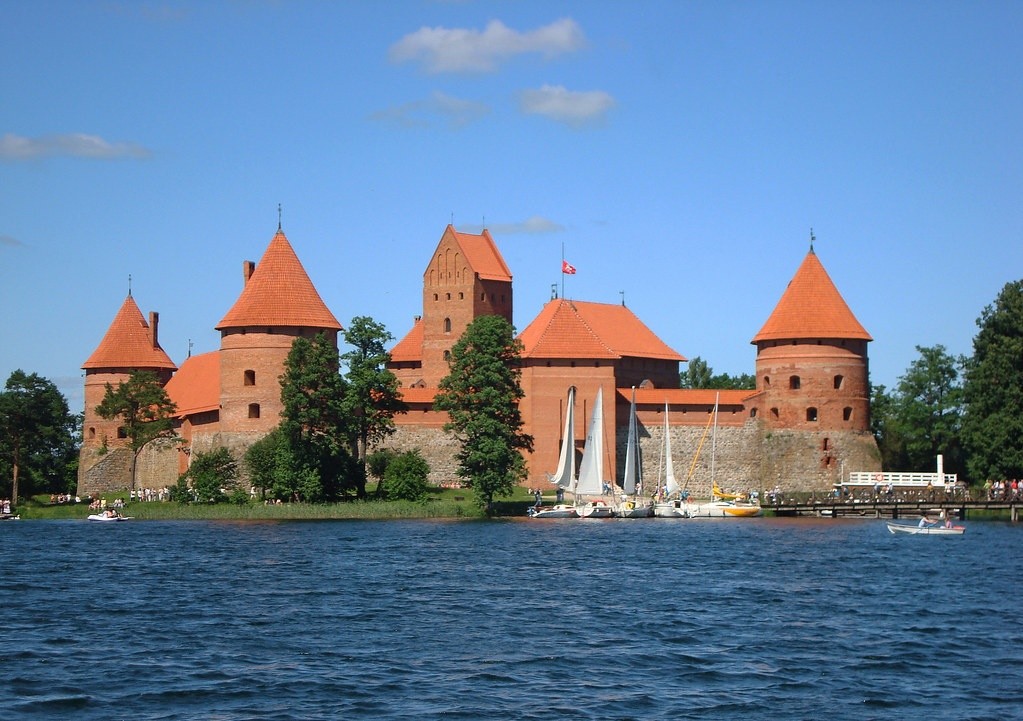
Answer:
[634,481,642,495]
[602,480,612,495]
[527,486,542,506]
[556,487,564,501]
[0,498,11,514]
[828,479,1023,529]
[75,495,81,502]
[651,482,694,503]
[719,485,782,505]
[265,498,282,506]
[50,493,71,504]
[250,485,257,499]
[88,495,126,518]
[130,487,172,501]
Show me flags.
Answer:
[811,231,816,240]
[562,261,576,274]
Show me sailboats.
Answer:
[526,384,762,518]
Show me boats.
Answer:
[886,521,966,535]
[87,511,129,522]
